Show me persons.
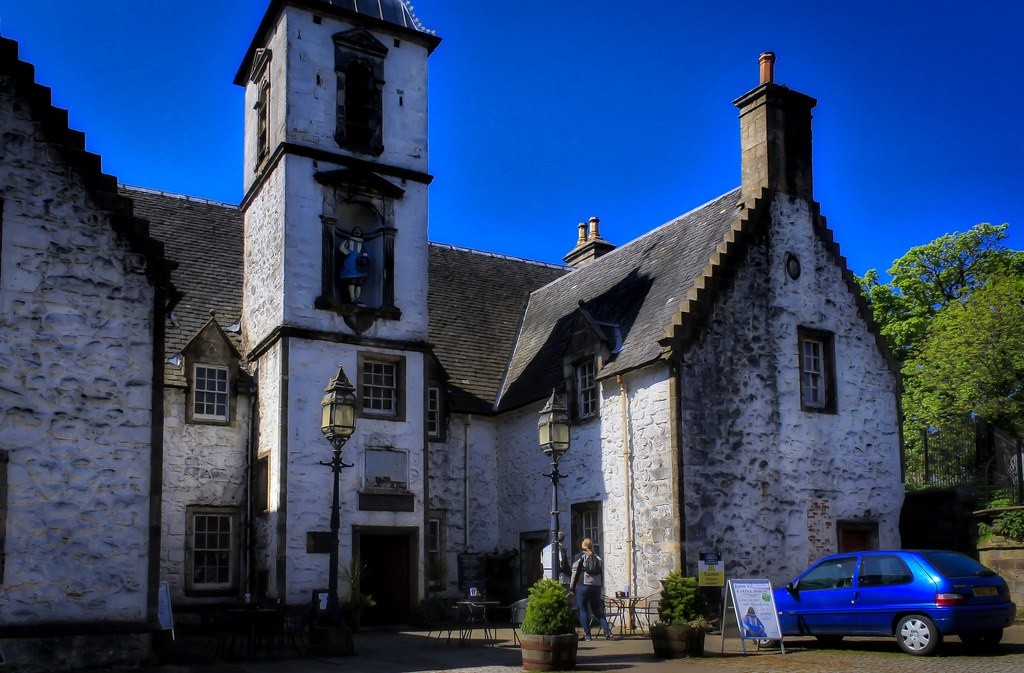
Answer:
[569,537,614,642]
[339,225,370,306]
[539,531,572,591]
[741,607,767,637]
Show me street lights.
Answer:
[537,387,572,582]
[309,363,355,657]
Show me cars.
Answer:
[752,549,1015,656]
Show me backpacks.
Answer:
[580,553,601,575]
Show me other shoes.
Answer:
[606,633,613,640]
[580,636,590,641]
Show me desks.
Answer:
[220,609,285,660]
[455,600,501,645]
[601,596,642,640]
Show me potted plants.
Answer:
[652,575,709,658]
[337,565,379,629]
[518,581,580,669]
[422,560,462,621]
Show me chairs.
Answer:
[420,599,525,647]
[891,561,903,583]
[595,592,663,639]
[864,563,882,586]
[197,597,313,664]
